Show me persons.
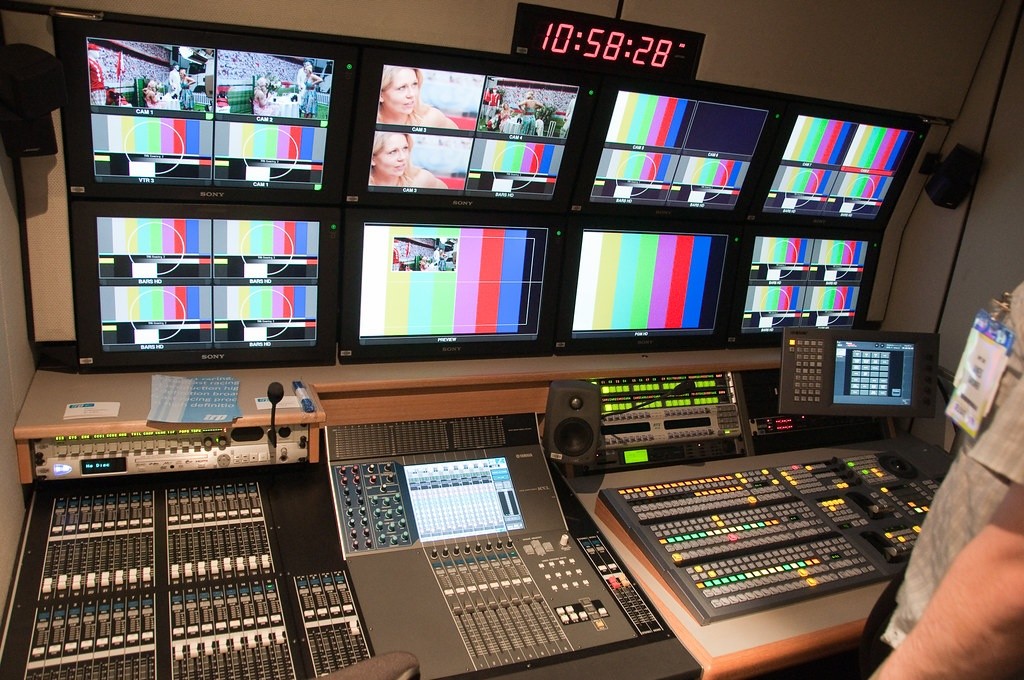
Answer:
[478,84,577,138]
[854,282,1024,680]
[87,43,323,119]
[369,131,448,189]
[392,237,457,272]
[376,65,459,129]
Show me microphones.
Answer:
[267,382,285,448]
[601,379,696,417]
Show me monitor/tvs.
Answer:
[404,457,524,542]
[49,16,937,407]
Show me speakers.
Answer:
[543,380,602,466]
[0,42,58,156]
[926,143,985,211]
